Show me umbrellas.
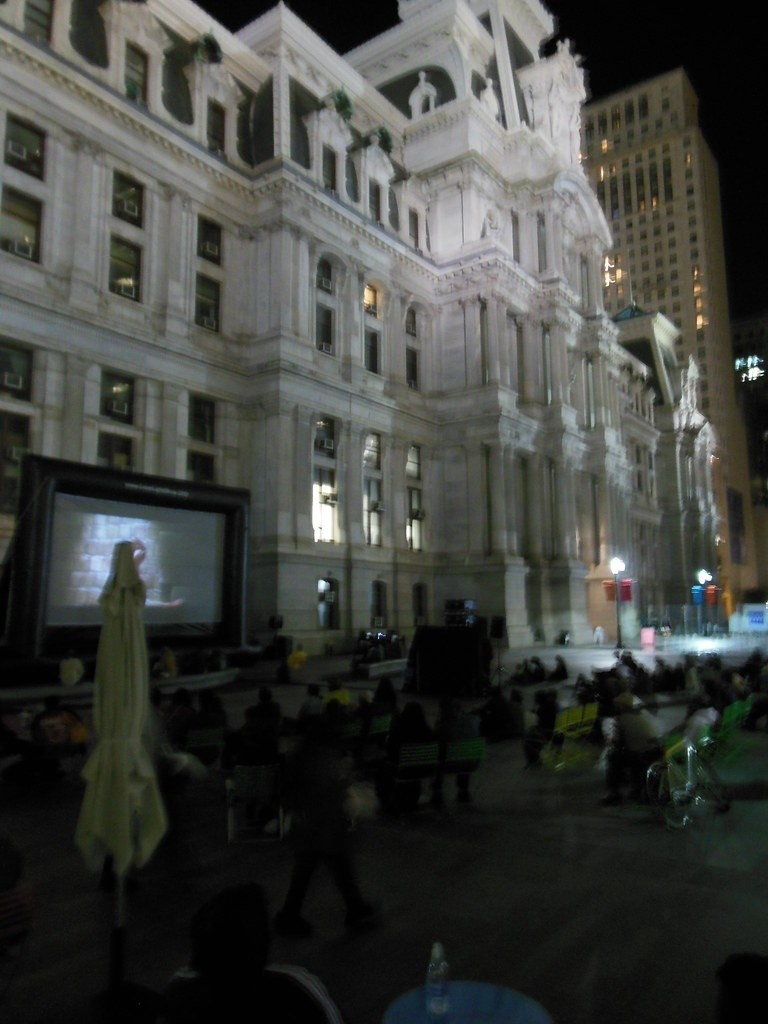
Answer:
[72,544,166,971]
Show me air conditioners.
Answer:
[374,616,389,629]
[369,304,376,313]
[113,401,127,418]
[10,447,31,463]
[6,140,27,162]
[318,277,331,291]
[9,240,32,258]
[116,280,136,299]
[410,509,425,521]
[414,616,427,626]
[320,494,338,504]
[119,199,138,217]
[409,380,419,390]
[371,499,385,514]
[201,316,215,330]
[321,438,334,450]
[2,370,24,389]
[408,323,416,334]
[319,342,332,354]
[202,242,218,256]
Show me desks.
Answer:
[383,981,553,1024]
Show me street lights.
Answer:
[698,568,712,638]
[610,557,628,649]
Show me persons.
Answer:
[477,652,767,809]
[1,684,225,798]
[228,673,478,935]
[1,829,37,969]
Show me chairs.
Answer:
[0,694,755,1024]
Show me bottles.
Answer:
[424,943,449,1019]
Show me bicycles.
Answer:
[643,720,731,833]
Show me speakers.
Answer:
[269,615,283,629]
